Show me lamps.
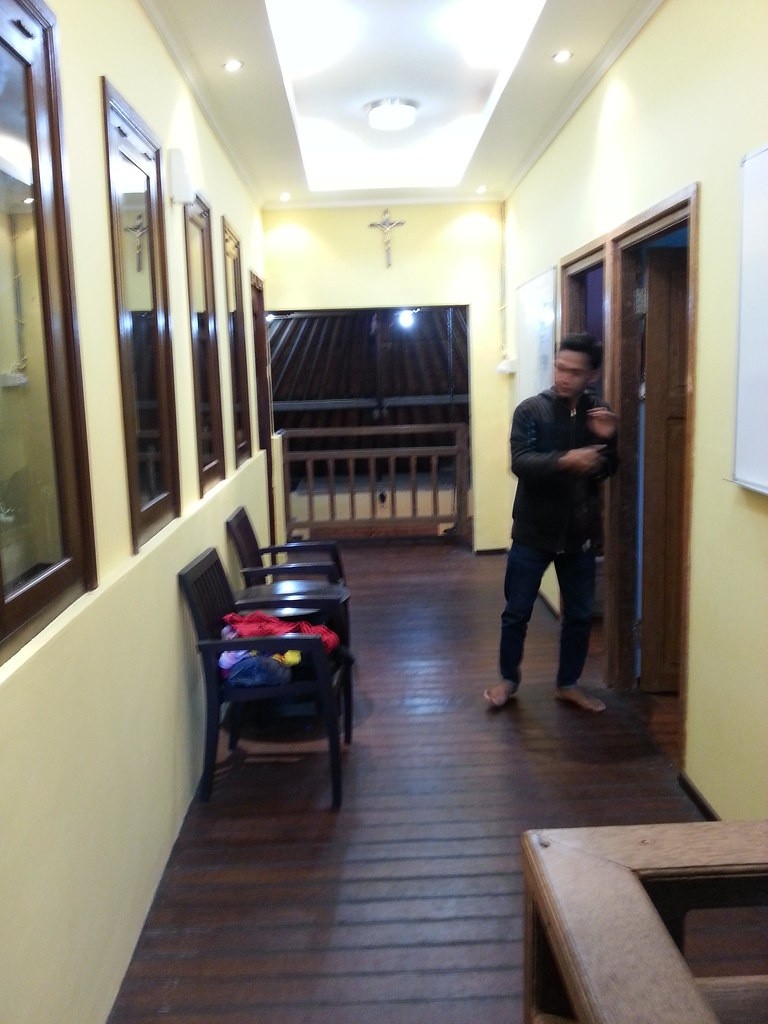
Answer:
[365,99,421,132]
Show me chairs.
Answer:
[177,547,356,813]
[226,505,351,651]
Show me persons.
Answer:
[483,332,616,712]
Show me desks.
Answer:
[233,579,351,717]
